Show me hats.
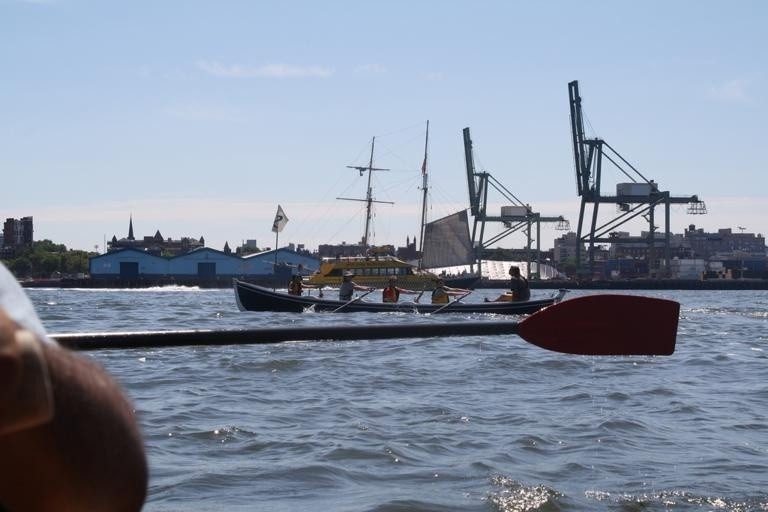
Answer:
[343,270,356,279]
[509,265,520,276]
[292,272,303,282]
[388,275,398,283]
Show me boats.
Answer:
[232,274,572,314]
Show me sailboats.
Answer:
[414,119,483,290]
[297,135,449,290]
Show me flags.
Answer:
[271,204,288,232]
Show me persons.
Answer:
[0,260,148,510]
[430,278,471,305]
[484,266,531,301]
[382,275,424,304]
[338,271,373,303]
[287,271,319,295]
